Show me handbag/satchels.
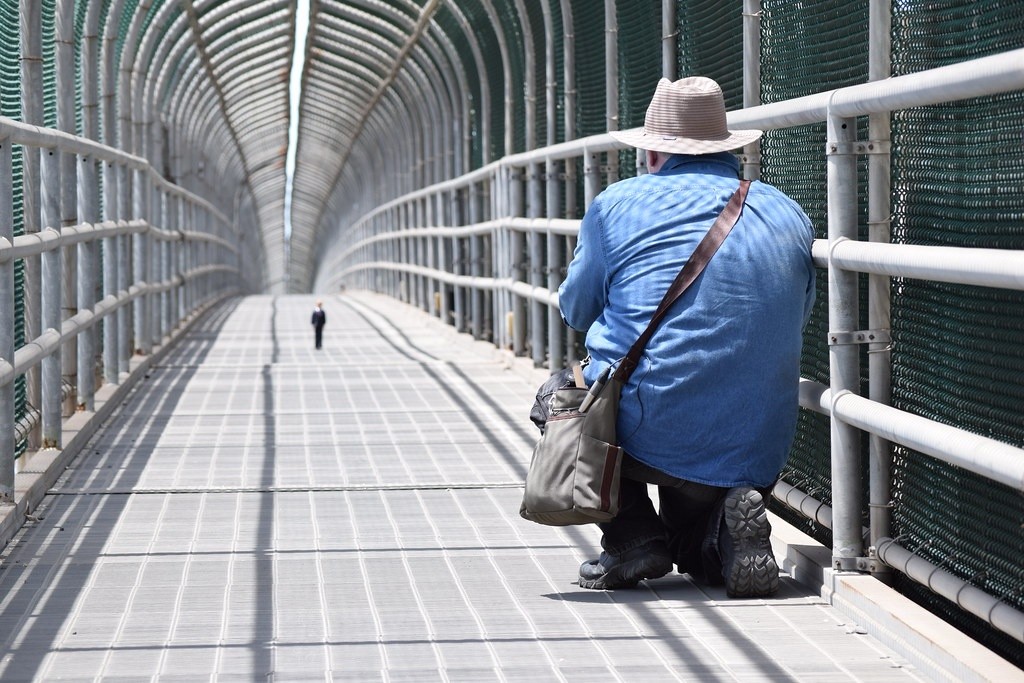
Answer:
[519,380,624,526]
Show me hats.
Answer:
[609,76,763,154]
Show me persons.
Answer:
[517,76,816,600]
[310,301,326,349]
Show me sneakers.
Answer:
[578,537,673,589]
[717,485,780,599]
